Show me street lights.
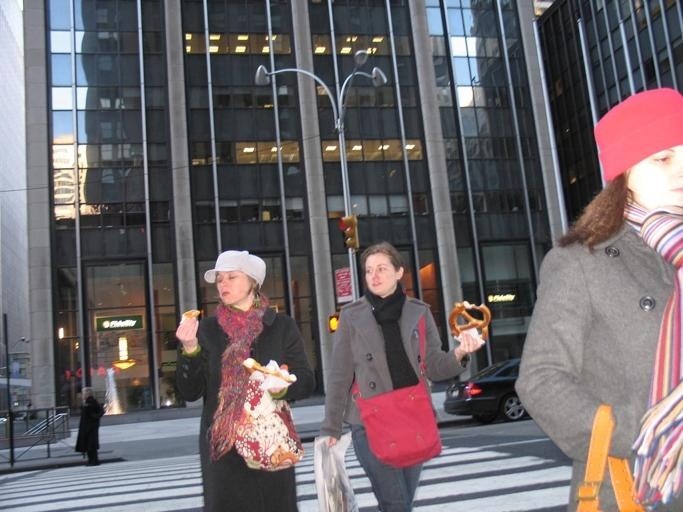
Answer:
[255,50,388,301]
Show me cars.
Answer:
[443,359,527,422]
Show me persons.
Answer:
[73,386,105,466]
[314,242,487,511]
[173,249,319,511]
[513,89,682,510]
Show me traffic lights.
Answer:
[340,216,359,251]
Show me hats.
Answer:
[593,87,683,185]
[203,249,267,290]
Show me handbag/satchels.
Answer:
[355,381,442,471]
[229,398,304,473]
[313,429,361,512]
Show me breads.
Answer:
[179,309,200,324]
[449,300,491,342]
[243,358,297,384]
